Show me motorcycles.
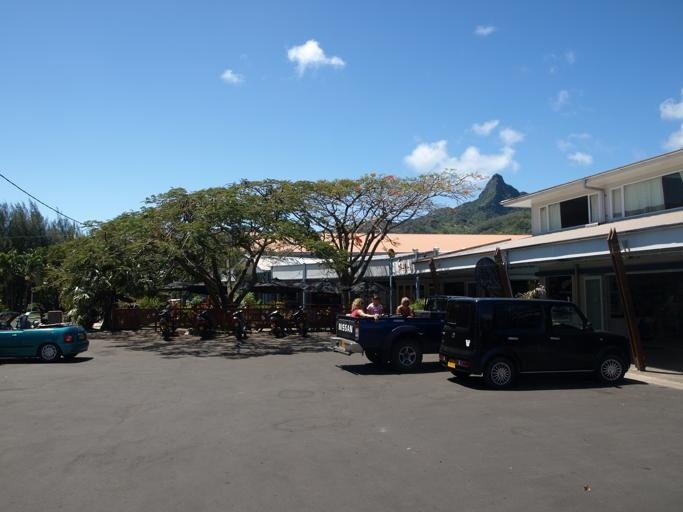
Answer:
[158,302,178,341]
[192,305,214,338]
[226,306,247,340]
[263,306,285,338]
[289,305,306,338]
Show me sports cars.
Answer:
[0,311,89,362]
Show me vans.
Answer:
[437,296,631,387]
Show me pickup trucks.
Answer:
[331,294,472,372]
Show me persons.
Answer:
[366,295,383,315]
[396,297,415,317]
[351,298,379,322]
